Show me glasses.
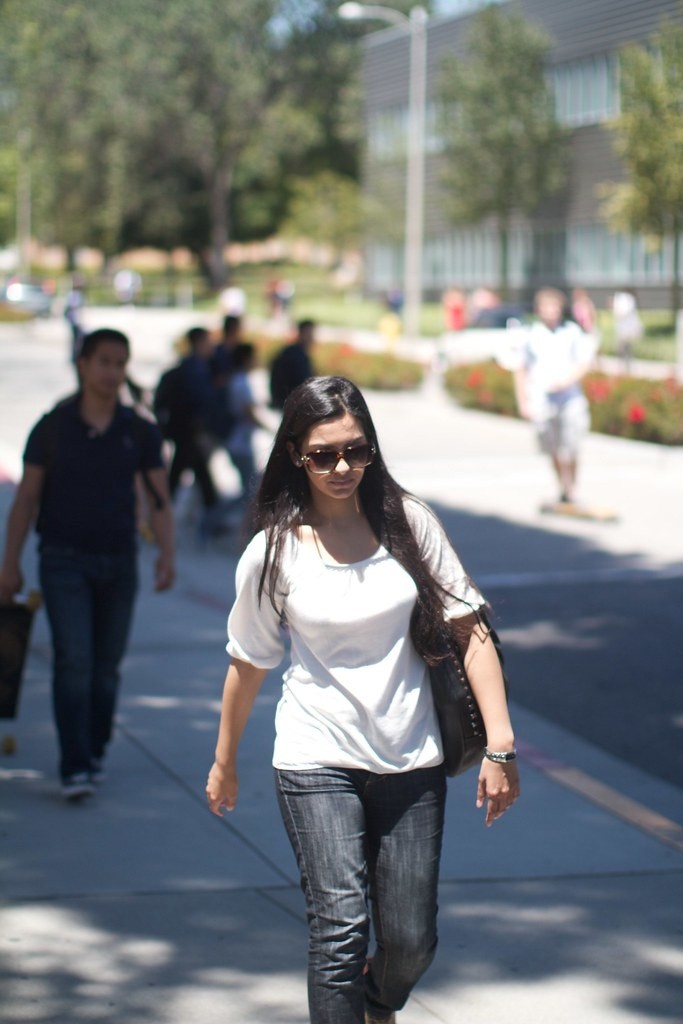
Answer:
[295,441,375,474]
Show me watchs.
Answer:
[485,748,518,763]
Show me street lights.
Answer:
[335,2,427,356]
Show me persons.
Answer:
[59,259,299,329]
[206,375,520,1024]
[384,262,646,379]
[0,328,176,800]
[152,316,319,536]
[514,286,600,505]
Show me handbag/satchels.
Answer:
[389,494,513,776]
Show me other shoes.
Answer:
[2,734,15,754]
[199,518,230,534]
[89,760,117,792]
[62,772,95,800]
[362,957,397,1024]
